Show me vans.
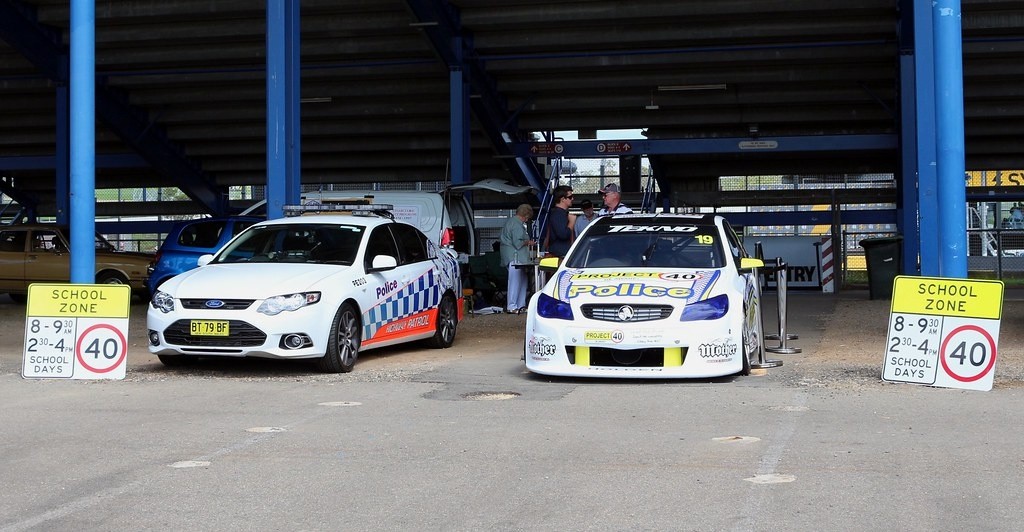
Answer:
[219,178,539,311]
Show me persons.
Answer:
[499,204,534,313]
[599,183,633,220]
[572,199,599,241]
[548,186,577,264]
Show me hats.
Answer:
[598,183,621,194]
[581,199,592,209]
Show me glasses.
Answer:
[565,194,574,200]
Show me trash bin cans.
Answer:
[859,236,902,299]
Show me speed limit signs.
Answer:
[880,275,1006,392]
[20,283,132,382]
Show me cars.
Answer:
[147,217,321,306]
[147,200,465,374]
[523,212,766,382]
[0,221,159,294]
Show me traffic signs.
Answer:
[596,141,632,153]
[529,143,564,155]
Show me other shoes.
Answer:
[508,308,527,314]
